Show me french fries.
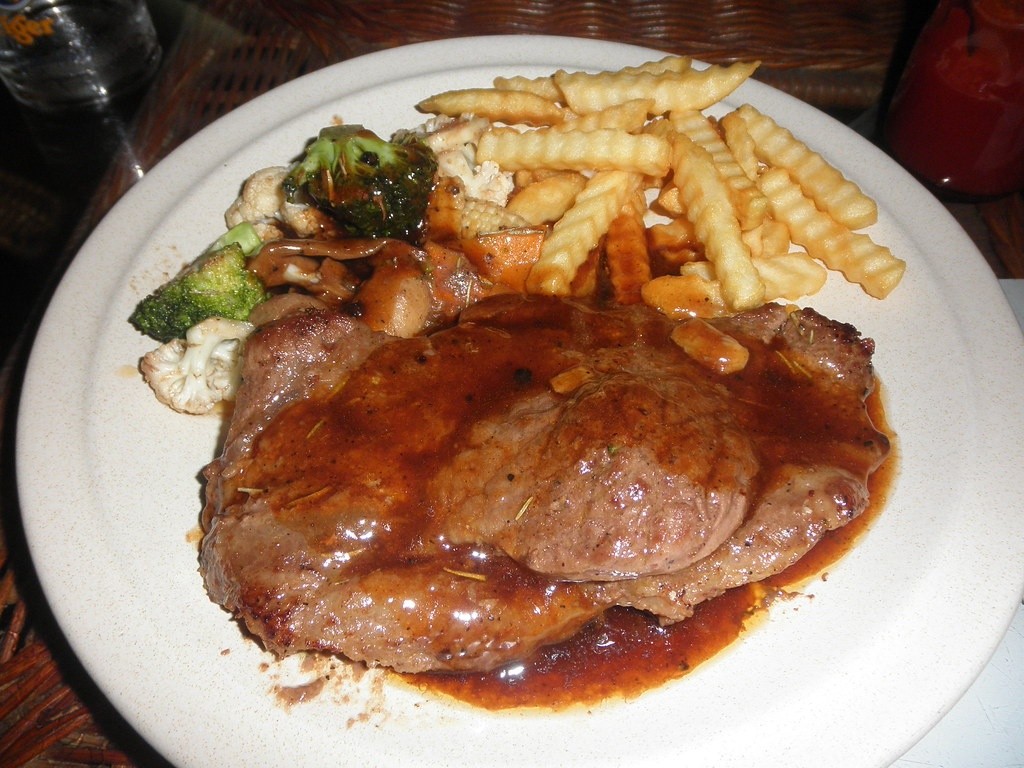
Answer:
[415,52,906,325]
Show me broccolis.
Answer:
[117,120,517,411]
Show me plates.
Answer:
[16,35,1024,768]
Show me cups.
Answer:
[874,1,1023,204]
[0,0,163,125]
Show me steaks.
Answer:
[197,290,891,675]
